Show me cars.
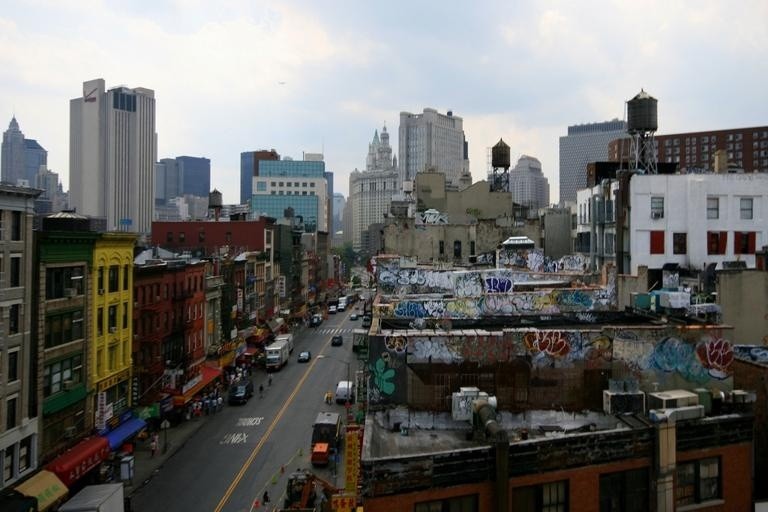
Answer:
[298,351,311,363]
[350,313,357,320]
[328,306,337,314]
[332,335,342,346]
[338,304,344,311]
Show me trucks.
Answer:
[339,296,348,309]
[264,341,289,370]
[276,333,294,353]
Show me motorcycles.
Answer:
[326,392,332,405]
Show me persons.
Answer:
[259,384,264,399]
[268,371,273,385]
[185,384,224,420]
[150,440,155,456]
[313,316,317,327]
[263,491,270,512]
[225,362,247,384]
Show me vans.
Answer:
[310,314,322,326]
[229,379,253,404]
[335,381,355,405]
[311,411,341,449]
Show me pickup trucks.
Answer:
[361,316,371,327]
